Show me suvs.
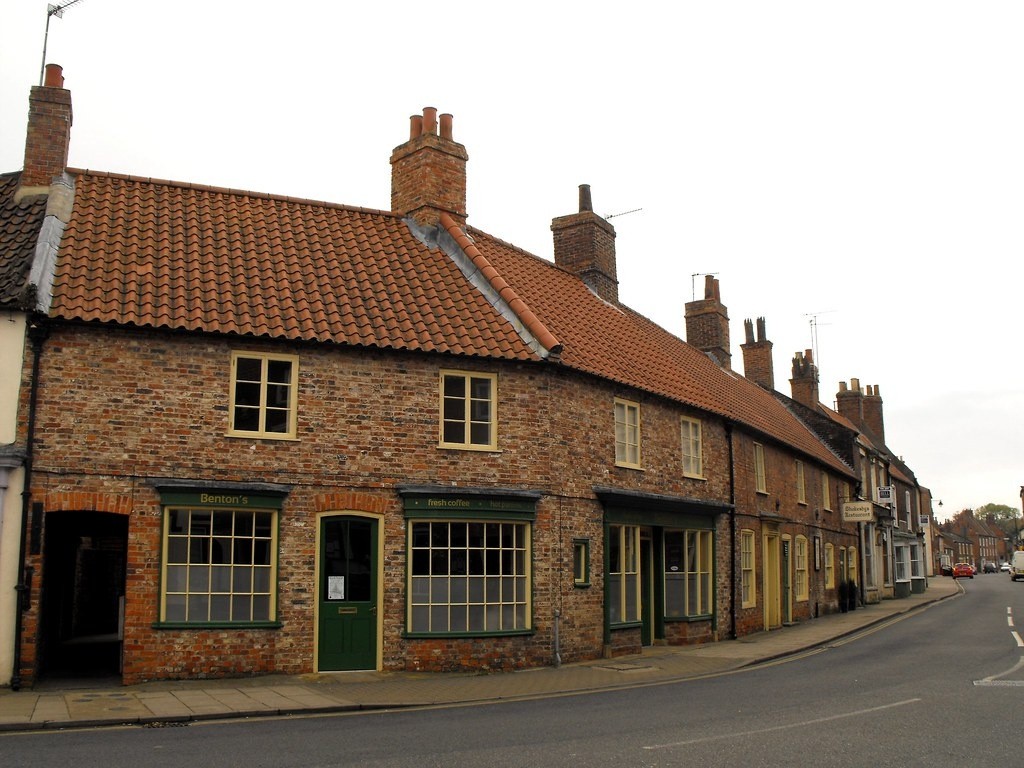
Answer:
[984,562,998,573]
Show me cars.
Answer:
[1001,562,1013,571]
[953,563,974,579]
[941,565,953,576]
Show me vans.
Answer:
[1008,551,1024,581]
